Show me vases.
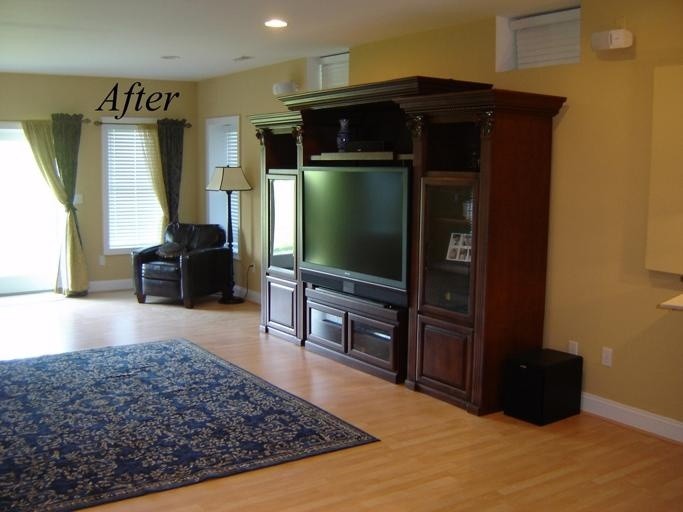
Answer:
[335,118,350,152]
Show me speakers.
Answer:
[273,80,299,95]
[591,29,633,49]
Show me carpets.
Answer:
[0,336,381,512]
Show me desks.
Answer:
[656,292,683,309]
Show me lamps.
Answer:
[203,165,253,305]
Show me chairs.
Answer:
[130,221,233,308]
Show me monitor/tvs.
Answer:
[297,167,408,310]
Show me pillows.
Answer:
[154,242,196,261]
